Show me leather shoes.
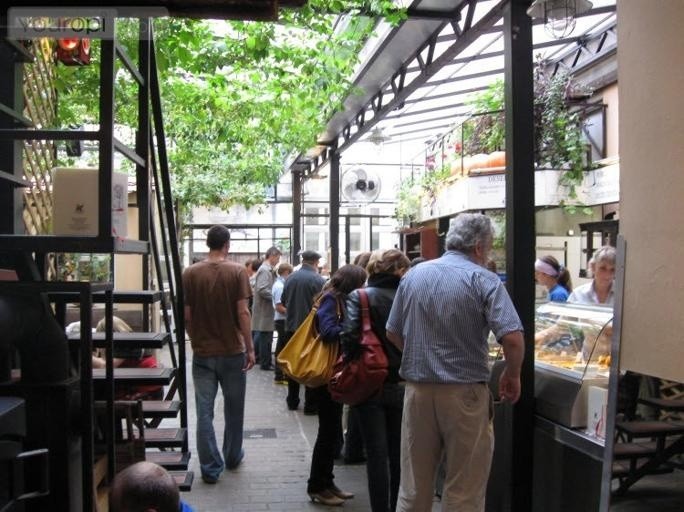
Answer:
[323,487,356,499]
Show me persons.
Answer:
[92,315,164,400]
[183,225,256,483]
[386,212,525,512]
[108,461,196,512]
[247,247,425,511]
[534,245,616,363]
[534,255,585,353]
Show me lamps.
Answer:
[526,0,593,39]
[53,34,92,66]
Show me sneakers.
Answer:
[287,396,319,416]
[201,472,218,484]
[254,357,276,371]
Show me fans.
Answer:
[340,164,381,206]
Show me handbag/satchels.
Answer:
[276,292,341,388]
[328,287,389,406]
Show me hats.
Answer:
[302,250,322,259]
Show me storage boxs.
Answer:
[52,167,129,239]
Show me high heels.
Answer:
[308,489,345,506]
